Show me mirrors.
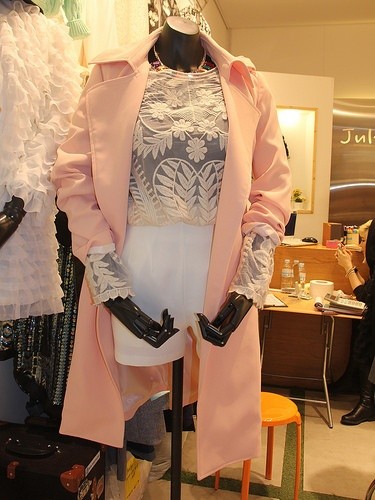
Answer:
[277,106,319,214]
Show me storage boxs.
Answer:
[344,233,359,245]
[323,222,343,245]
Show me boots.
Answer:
[342,379,375,424]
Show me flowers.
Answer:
[291,188,306,203]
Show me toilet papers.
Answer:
[309,280,335,301]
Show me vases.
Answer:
[294,203,304,210]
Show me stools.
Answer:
[214,391,302,500]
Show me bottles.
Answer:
[299,263,306,289]
[281,259,293,293]
[292,260,300,290]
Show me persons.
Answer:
[53,15,293,481]
[0,0,86,392]
[324,218,375,425]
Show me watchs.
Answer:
[353,266,358,273]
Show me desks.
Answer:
[261,290,364,428]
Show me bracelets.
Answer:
[344,269,356,278]
